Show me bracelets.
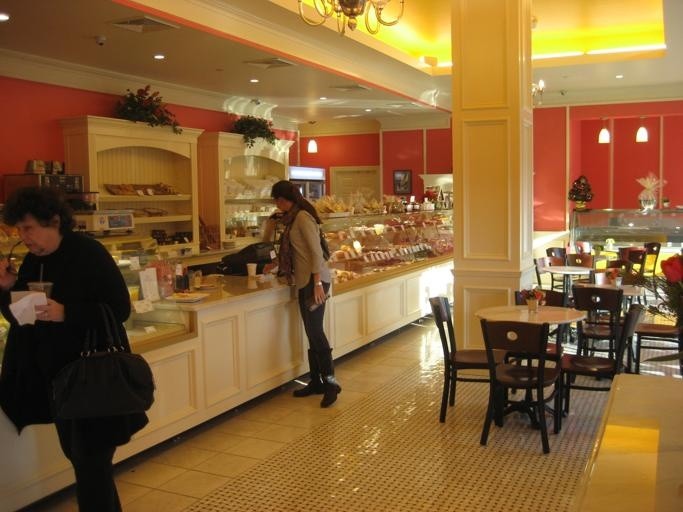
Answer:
[312,279,325,287]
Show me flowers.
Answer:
[567,175,594,201]
[112,82,186,136]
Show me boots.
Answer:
[294,348,325,397]
[314,347,341,407]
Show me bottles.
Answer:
[174,262,201,292]
[152,230,192,244]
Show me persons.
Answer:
[261,178,344,410]
[1,185,131,512]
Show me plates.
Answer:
[126,208,168,217]
[223,240,238,250]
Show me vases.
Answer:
[575,201,585,208]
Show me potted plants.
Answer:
[660,196,671,208]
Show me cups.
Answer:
[26,282,53,299]
[246,263,257,278]
[247,278,258,289]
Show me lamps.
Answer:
[597,116,649,145]
[297,0,404,38]
[307,120,318,153]
[529,77,546,98]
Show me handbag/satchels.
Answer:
[50,303,155,421]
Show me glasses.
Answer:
[6,240,24,275]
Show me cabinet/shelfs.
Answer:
[263,210,452,360]
[59,116,295,257]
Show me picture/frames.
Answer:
[392,170,412,194]
